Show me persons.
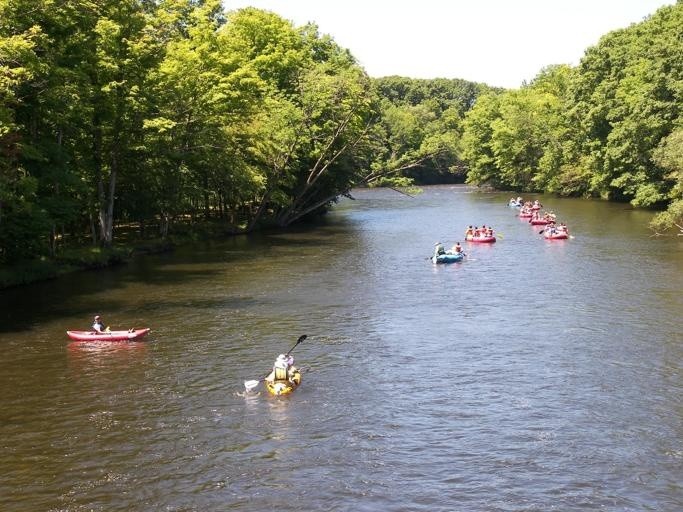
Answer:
[464,224,493,237]
[92,314,111,335]
[507,195,569,235]
[433,241,445,256]
[272,352,295,368]
[454,241,464,253]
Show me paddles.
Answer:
[266,335,309,378]
[494,233,502,237]
[540,229,545,234]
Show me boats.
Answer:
[432,248,463,264]
[530,217,556,225]
[544,224,568,240]
[510,201,521,207]
[533,207,540,210]
[65,340,148,353]
[265,363,301,397]
[519,213,533,217]
[522,205,533,210]
[465,234,496,243]
[65,328,150,340]
